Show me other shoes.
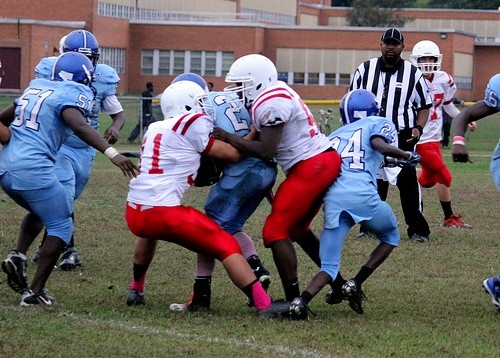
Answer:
[126,288,145,307]
[356,232,368,238]
[325,278,350,304]
[184,288,212,311]
[412,234,429,244]
[342,278,364,314]
[289,298,309,320]
[257,300,292,318]
[242,257,272,307]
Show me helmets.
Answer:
[161,80,205,119]
[410,40,442,69]
[52,51,95,83]
[340,89,378,125]
[171,72,207,93]
[59,30,99,71]
[223,53,277,112]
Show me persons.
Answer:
[441,96,477,147]
[125,52,346,319]
[451,74,500,308]
[289,89,422,319]
[410,39,473,230]
[349,29,471,243]
[126,81,158,143]
[0,30,141,308]
[207,82,214,92]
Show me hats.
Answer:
[381,28,404,45]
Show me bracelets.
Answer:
[414,125,423,135]
[453,135,465,146]
[104,147,120,160]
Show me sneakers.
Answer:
[59,246,82,270]
[444,213,472,229]
[1,249,28,295]
[482,274,500,308]
[30,247,40,265]
[19,287,55,307]
